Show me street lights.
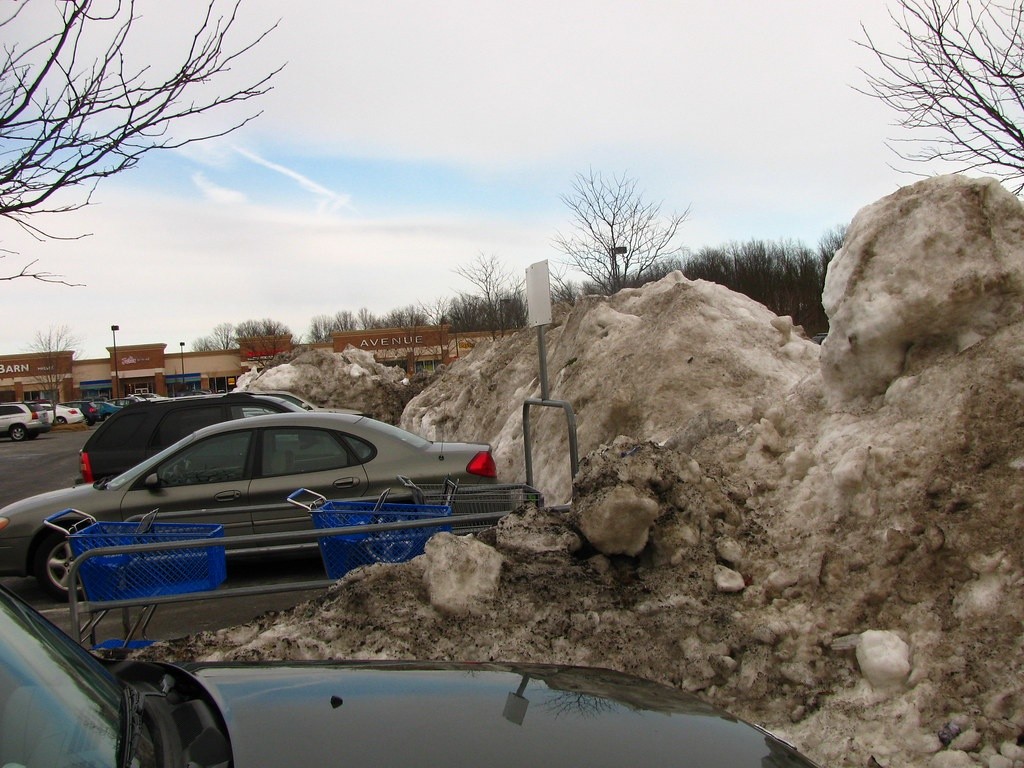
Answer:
[180,342,185,390]
[111,325,119,398]
[611,247,626,293]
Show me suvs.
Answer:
[79,390,369,483]
[0,402,51,441]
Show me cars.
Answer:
[40,393,162,425]
[0,582,820,768]
[0,411,498,602]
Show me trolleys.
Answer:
[397,475,544,536]
[286,487,454,582]
[43,507,228,650]
